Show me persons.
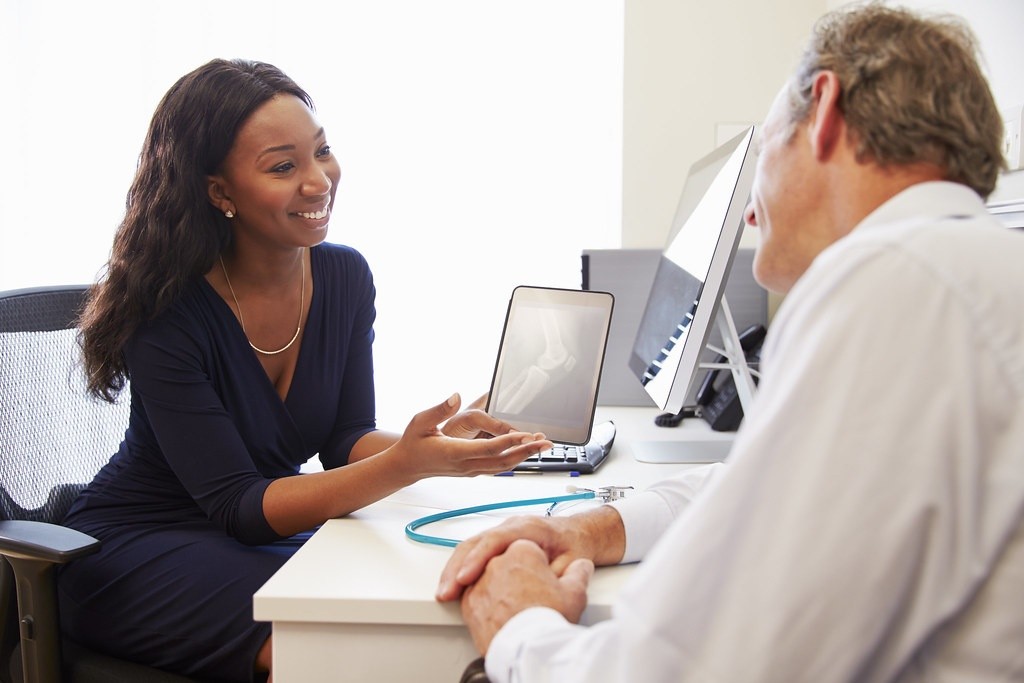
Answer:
[436,0,1023,683]
[53,59,553,683]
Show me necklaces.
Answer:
[218,247,305,354]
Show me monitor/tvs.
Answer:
[629,125,762,463]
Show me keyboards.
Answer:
[509,419,616,472]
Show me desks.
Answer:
[251,406,739,682]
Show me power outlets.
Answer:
[996,103,1024,173]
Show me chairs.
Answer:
[1,281,219,682]
